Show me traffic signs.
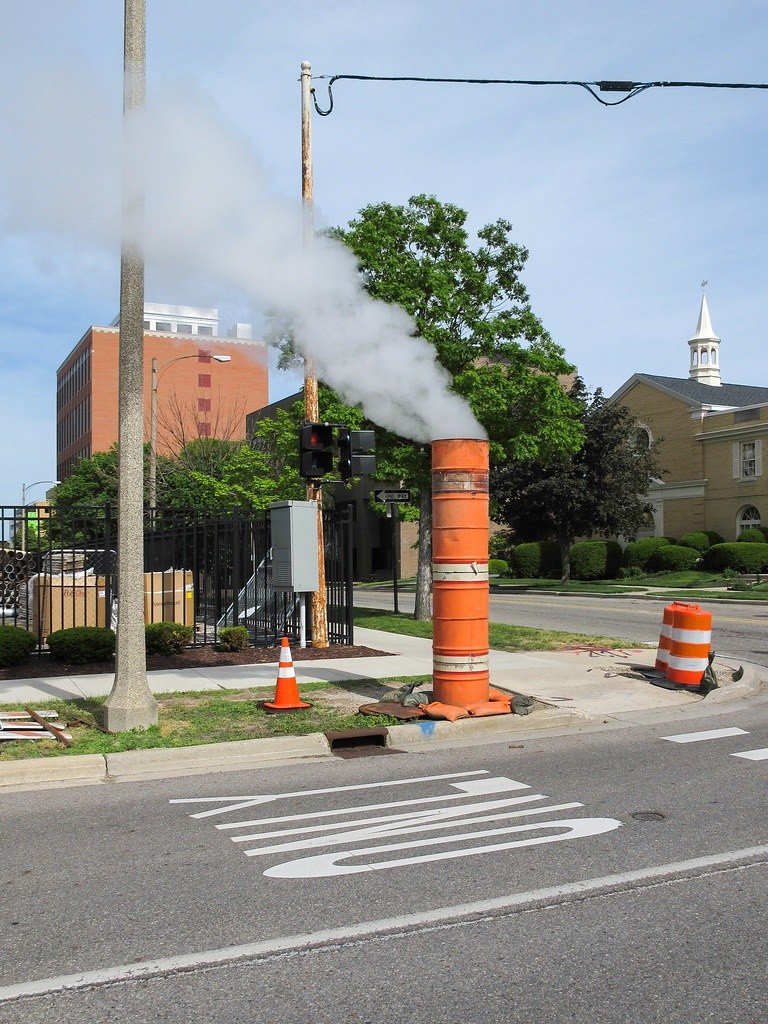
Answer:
[374,488,412,503]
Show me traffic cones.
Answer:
[262,637,314,714]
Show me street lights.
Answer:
[149,355,232,531]
[21,481,62,554]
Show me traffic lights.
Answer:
[340,428,377,481]
[301,424,335,479]
